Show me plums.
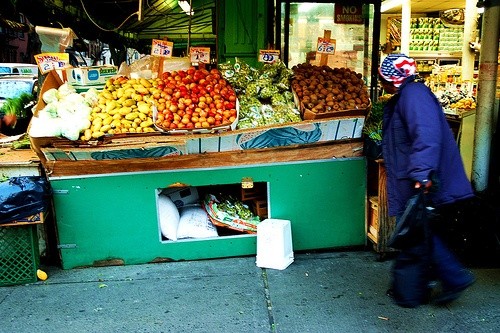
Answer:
[153,66,235,128]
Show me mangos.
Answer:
[80,76,162,141]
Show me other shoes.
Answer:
[386,288,418,309]
[432,270,478,306]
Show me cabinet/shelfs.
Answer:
[43,145,365,269]
[370,0,500,255]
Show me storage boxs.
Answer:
[203,182,268,233]
[0,226,40,286]
[162,188,199,209]
[0,63,118,101]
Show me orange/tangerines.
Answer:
[450,98,476,108]
[292,62,370,113]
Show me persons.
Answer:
[376,53,477,308]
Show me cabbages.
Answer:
[28,83,100,141]
